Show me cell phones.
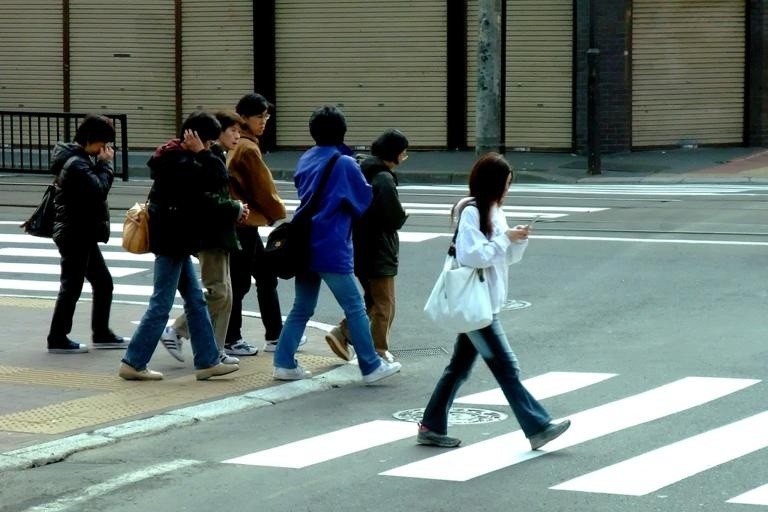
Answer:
[524,215,541,227]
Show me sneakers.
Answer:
[47,336,90,354]
[119,361,164,382]
[416,422,462,448]
[363,350,404,384]
[194,336,259,381]
[92,331,134,347]
[272,365,313,381]
[157,323,186,363]
[325,327,351,361]
[528,419,572,451]
[263,335,308,352]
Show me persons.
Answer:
[324,129,410,364]
[116,110,239,382]
[270,106,402,384]
[45,113,133,355]
[414,151,571,452]
[224,92,308,356]
[159,108,250,365]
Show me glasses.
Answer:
[252,113,271,120]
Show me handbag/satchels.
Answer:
[18,156,81,239]
[422,204,494,335]
[262,153,340,280]
[121,200,153,255]
